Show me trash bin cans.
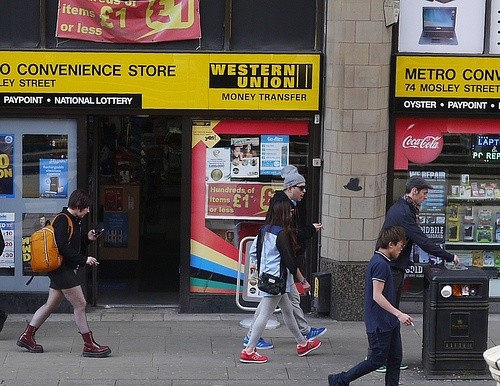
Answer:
[422,264,490,373]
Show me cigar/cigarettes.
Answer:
[411,322,414,327]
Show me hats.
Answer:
[407,175,433,190]
[281,164,306,188]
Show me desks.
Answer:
[97,183,139,264]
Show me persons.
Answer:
[328,225,415,386]
[15,189,112,358]
[242,164,328,350]
[35,216,47,231]
[364,175,460,372]
[232,143,253,162]
[239,200,322,364]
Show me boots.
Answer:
[78,331,111,356]
[16,320,43,353]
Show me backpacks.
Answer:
[30,213,74,273]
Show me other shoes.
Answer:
[328,374,338,386]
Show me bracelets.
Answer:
[302,279,307,284]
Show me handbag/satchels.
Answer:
[258,274,283,294]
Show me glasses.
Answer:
[292,185,307,191]
[398,242,406,250]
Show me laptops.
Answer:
[418,6,458,45]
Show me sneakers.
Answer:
[400,362,409,370]
[365,356,387,371]
[239,347,268,363]
[297,340,321,357]
[243,336,273,349]
[304,327,327,341]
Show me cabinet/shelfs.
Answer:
[445,198,500,267]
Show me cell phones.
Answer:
[93,228,104,235]
